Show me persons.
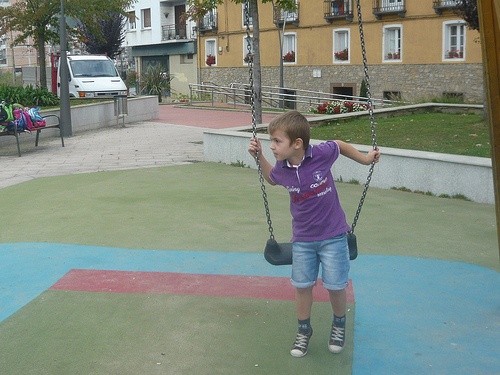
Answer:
[248,110,381,358]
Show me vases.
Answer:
[348,107,353,112]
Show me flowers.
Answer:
[448,50,463,58]
[384,52,400,60]
[282,51,295,63]
[344,101,353,107]
[334,48,348,61]
[206,54,215,66]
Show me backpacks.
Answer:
[0,101,46,133]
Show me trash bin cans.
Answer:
[114,95,128,129]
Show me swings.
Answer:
[244,0,378,266]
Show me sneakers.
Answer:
[290,327,313,357]
[328,321,345,354]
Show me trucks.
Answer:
[51,52,128,99]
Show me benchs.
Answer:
[0,114,64,157]
